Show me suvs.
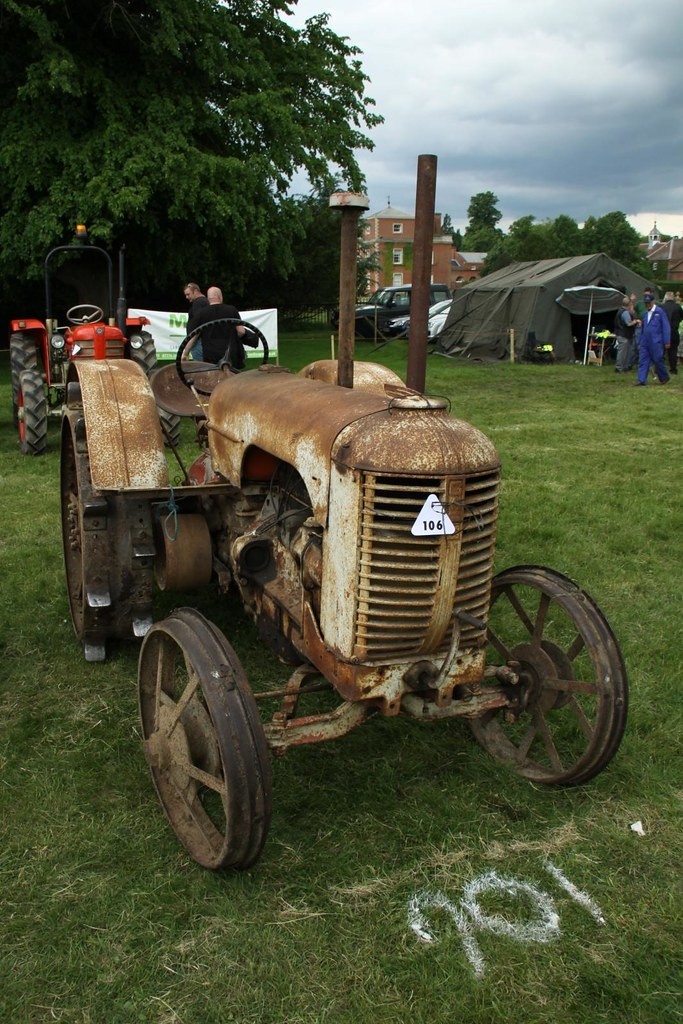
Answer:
[332,283,452,342]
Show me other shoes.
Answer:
[633,381,645,385]
[658,376,670,384]
[615,369,620,373]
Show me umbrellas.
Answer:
[555,285,629,365]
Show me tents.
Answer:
[435,251,662,366]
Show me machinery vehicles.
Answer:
[6,223,158,455]
[60,155,627,876]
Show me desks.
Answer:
[587,331,619,366]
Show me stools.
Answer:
[149,360,235,416]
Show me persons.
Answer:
[183,282,210,361]
[632,294,671,386]
[188,286,246,371]
[614,287,683,374]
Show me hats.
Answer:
[642,294,654,302]
